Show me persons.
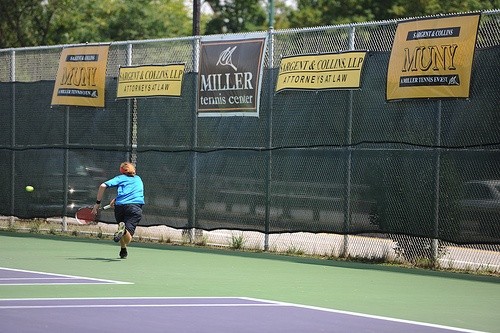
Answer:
[91,163,145,258]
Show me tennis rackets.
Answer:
[74,204,114,224]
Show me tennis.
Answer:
[25,186,34,192]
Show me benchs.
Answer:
[134,170,378,233]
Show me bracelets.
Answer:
[96,199,101,204]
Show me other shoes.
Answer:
[113,221,125,243]
[119,248,127,258]
